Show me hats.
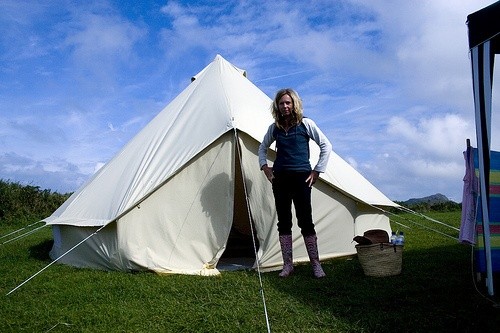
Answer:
[352,228,389,244]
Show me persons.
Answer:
[258,87,333,278]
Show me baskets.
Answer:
[355,241,403,277]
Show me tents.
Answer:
[38,51,404,279]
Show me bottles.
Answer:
[397,232,405,245]
[390,232,398,244]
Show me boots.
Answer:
[277,234,294,278]
[304,234,326,278]
